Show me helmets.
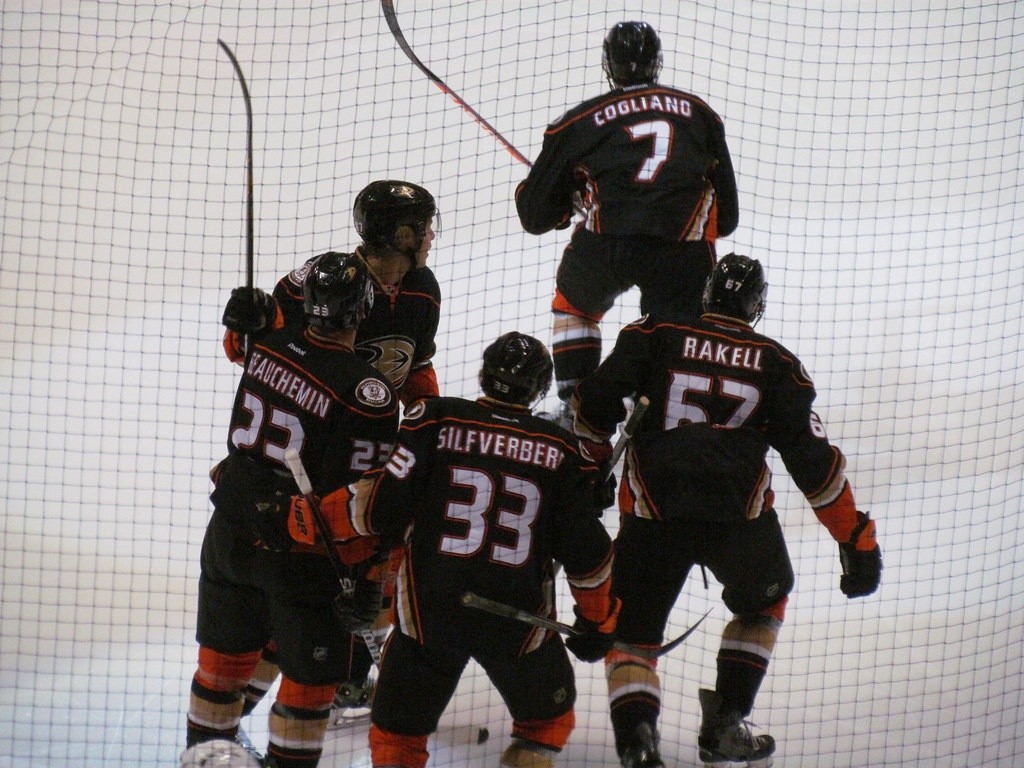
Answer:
[704,253,767,324]
[478,331,552,409]
[601,21,664,89]
[355,179,435,258]
[303,251,375,331]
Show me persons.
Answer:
[518,19,743,408]
[572,252,884,768]
[179,178,621,768]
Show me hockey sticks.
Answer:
[217,38,255,363]
[381,0,588,219]
[459,590,715,660]
[551,395,650,578]
[284,447,490,748]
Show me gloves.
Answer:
[334,555,387,631]
[222,285,278,338]
[579,436,613,467]
[256,492,320,552]
[838,509,882,598]
[566,592,622,662]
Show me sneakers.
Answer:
[613,702,665,768]
[234,725,266,765]
[697,688,776,768]
[325,677,378,729]
[533,396,576,436]
